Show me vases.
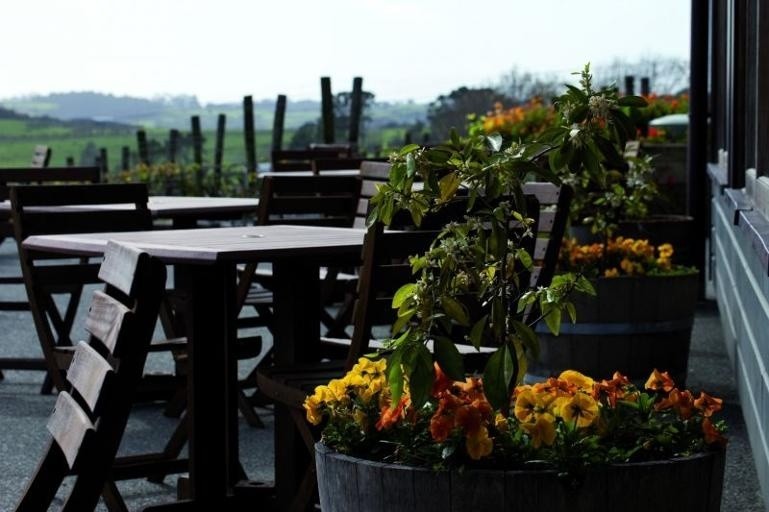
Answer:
[313,441,725,512]
